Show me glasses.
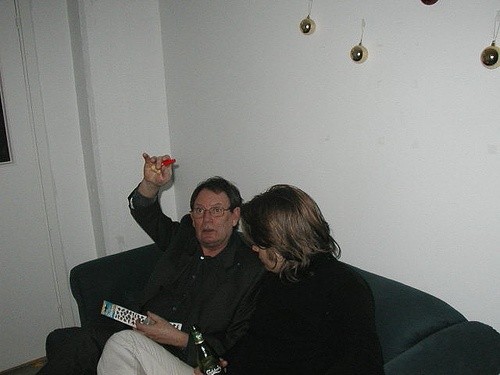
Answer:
[189,207,230,219]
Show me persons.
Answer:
[46,153,266,375]
[97,184,385,375]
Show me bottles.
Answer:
[190,324,226,375]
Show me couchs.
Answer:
[70,244,500,375]
